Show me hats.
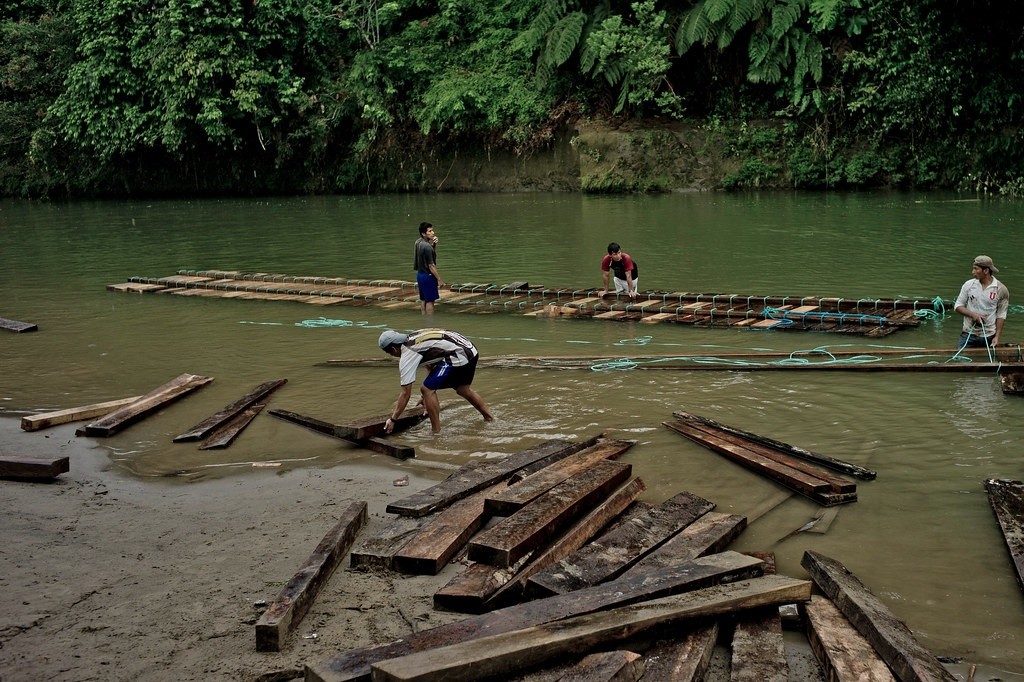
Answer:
[379,331,407,349]
[974,255,999,273]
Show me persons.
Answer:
[379,328,494,435]
[598,243,638,300]
[414,222,444,317]
[952,254,1010,350]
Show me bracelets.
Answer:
[389,417,396,423]
